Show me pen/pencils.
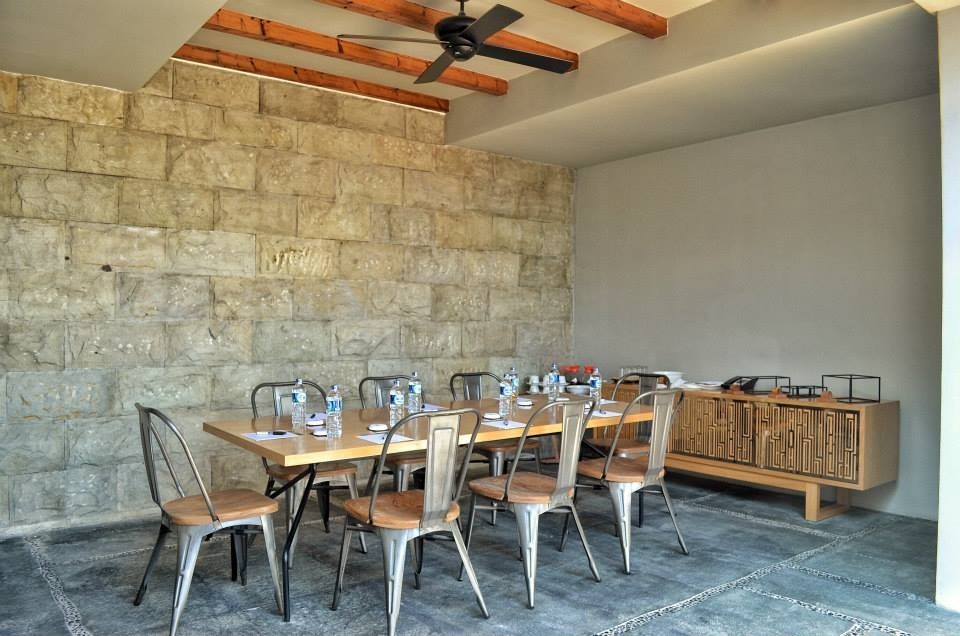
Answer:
[311,412,317,418]
[504,419,508,425]
[257,432,286,435]
[383,432,387,439]
[421,404,424,408]
[600,409,605,413]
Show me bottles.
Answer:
[388,380,405,429]
[291,378,307,434]
[589,368,602,411]
[498,374,512,416]
[508,367,520,400]
[326,384,342,438]
[548,362,559,400]
[407,371,422,415]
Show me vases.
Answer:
[568,384,589,396]
[446,44,475,63]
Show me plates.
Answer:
[683,379,724,390]
[560,383,570,386]
[526,391,541,394]
[539,383,548,386]
[526,382,543,386]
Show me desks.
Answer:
[201,393,678,622]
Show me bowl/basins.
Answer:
[567,385,590,394]
[652,371,682,388]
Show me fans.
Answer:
[336,0,574,85]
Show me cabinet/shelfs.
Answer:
[597,381,900,518]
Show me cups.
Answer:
[527,374,567,395]
[619,365,648,380]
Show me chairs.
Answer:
[358,374,464,533]
[560,387,686,574]
[251,379,368,564]
[330,409,491,636]
[446,372,544,522]
[458,396,602,612]
[581,372,671,529]
[133,403,283,635]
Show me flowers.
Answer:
[561,364,593,385]
